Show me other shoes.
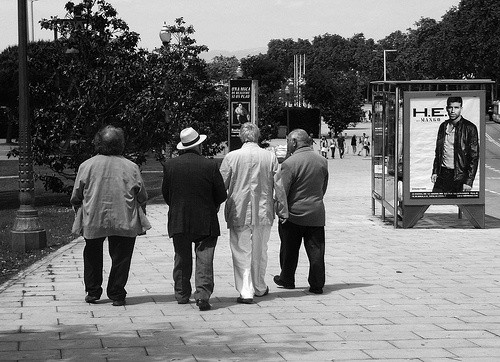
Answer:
[113,299,126,306]
[85,296,100,302]
[274,275,295,289]
[237,297,253,303]
[178,299,190,304]
[254,286,269,297]
[196,298,212,311]
[309,286,323,294]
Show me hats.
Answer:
[177,128,207,149]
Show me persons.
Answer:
[69,125,152,306]
[430,96,479,193]
[309,132,370,159]
[273,129,329,293]
[218,122,289,304]
[161,127,227,311]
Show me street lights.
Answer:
[158,20,172,159]
[285,85,290,135]
[235,66,243,98]
